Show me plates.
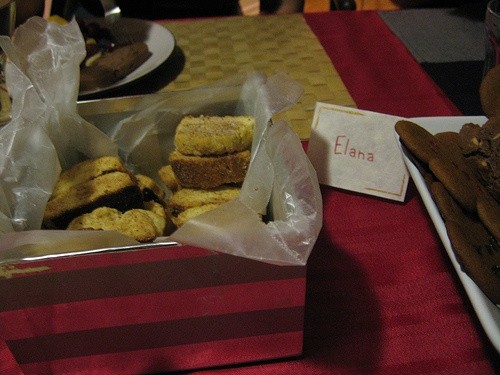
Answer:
[14,19,176,98]
[395,116,500,352]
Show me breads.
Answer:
[39,113,255,242]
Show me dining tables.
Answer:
[0,0,500,375]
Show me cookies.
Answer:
[394,66,500,303]
